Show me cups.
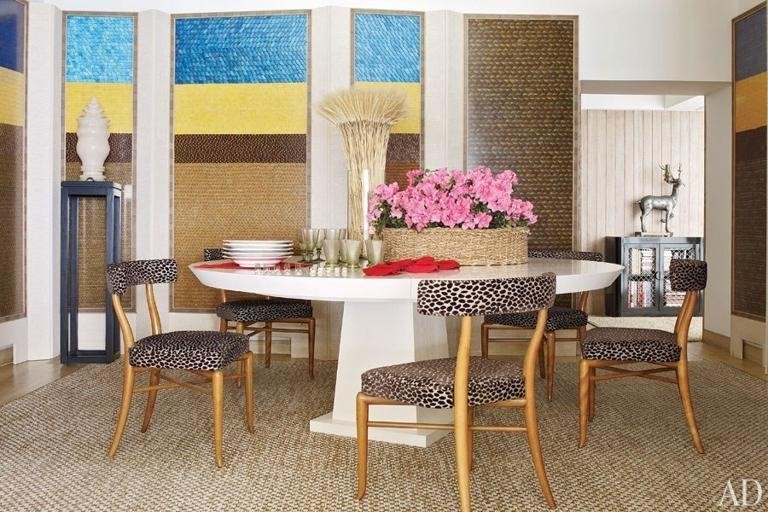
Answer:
[254,263,302,276]
[322,238,363,268]
[364,240,385,267]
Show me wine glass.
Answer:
[301,226,345,263]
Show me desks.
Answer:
[58,180,123,366]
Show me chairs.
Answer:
[202,247,319,383]
[353,270,560,512]
[106,257,253,468]
[481,248,605,405]
[575,258,710,449]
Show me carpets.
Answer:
[0,360,768,511]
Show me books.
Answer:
[628,280,653,308]
[664,249,692,271]
[629,248,654,274]
[664,278,686,307]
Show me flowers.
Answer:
[368,162,538,230]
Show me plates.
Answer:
[222,240,294,268]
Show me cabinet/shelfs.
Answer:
[605,235,704,315]
